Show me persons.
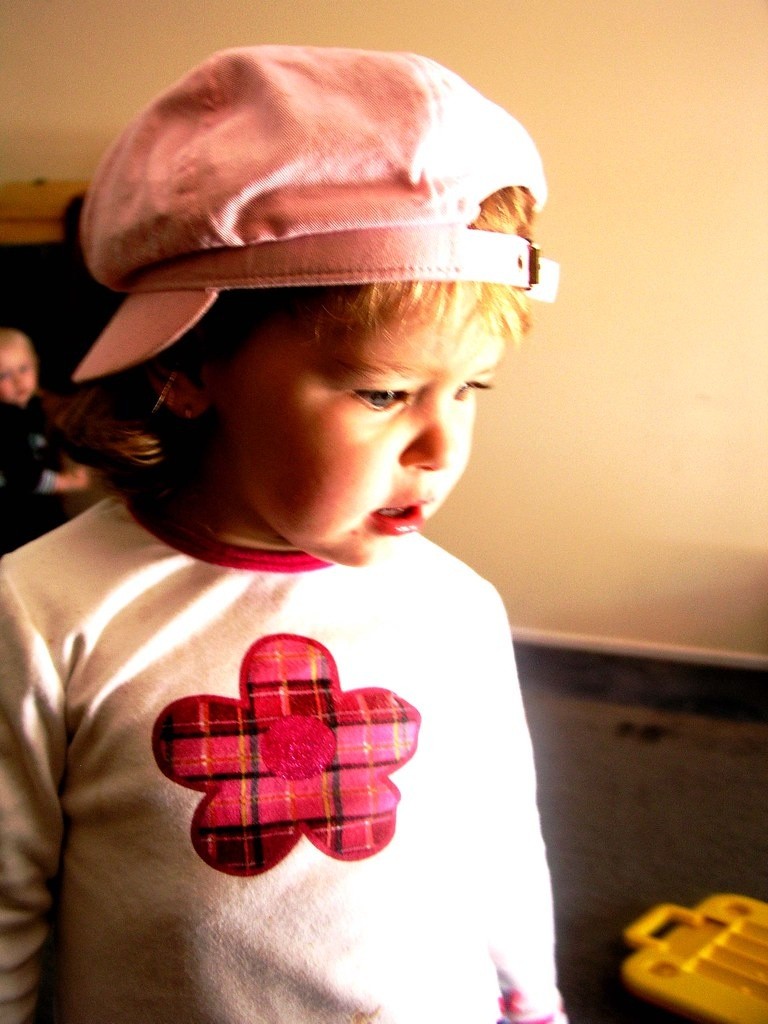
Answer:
[3,47,574,1024]
[1,328,102,553]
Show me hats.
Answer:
[68,46,561,381]
